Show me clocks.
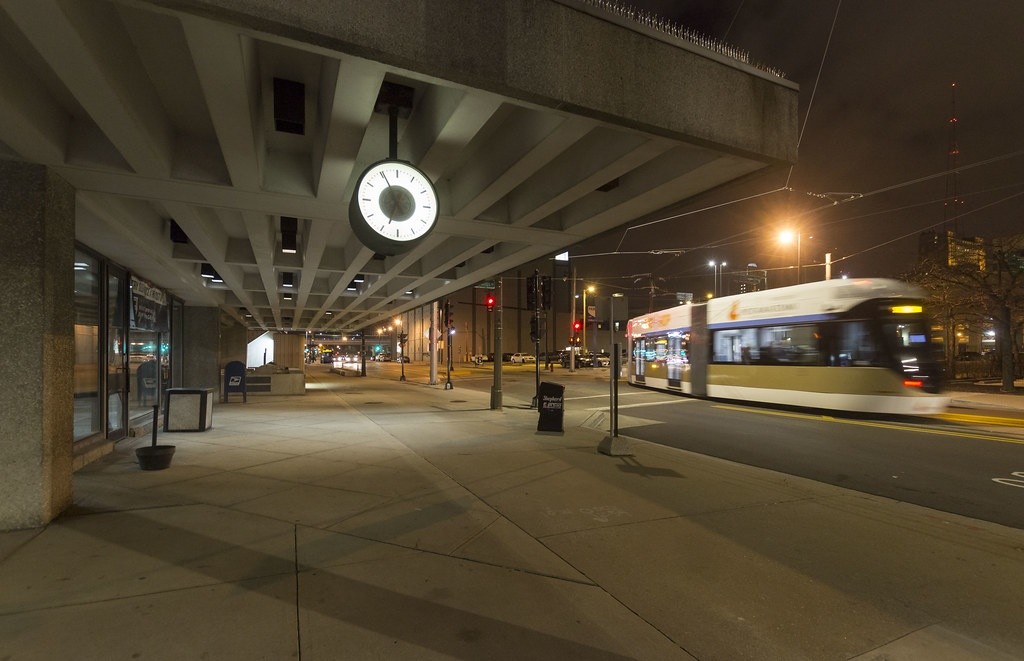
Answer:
[348,80,440,257]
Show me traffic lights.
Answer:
[444,299,453,329]
[401,333,408,344]
[487,295,495,311]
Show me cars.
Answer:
[369,353,392,362]
[537,350,611,367]
[510,353,536,364]
[321,351,335,363]
[396,356,410,364]
[471,352,514,365]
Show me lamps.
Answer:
[346,274,365,291]
[201,263,223,283]
[170,220,192,244]
[280,216,298,254]
[284,293,292,301]
[283,271,293,288]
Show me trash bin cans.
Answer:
[224,361,247,403]
[137,360,157,408]
[536,381,565,432]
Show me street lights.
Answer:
[709,261,717,298]
[377,329,383,344]
[720,261,727,297]
[781,229,803,284]
[609,292,624,344]
[394,319,407,381]
[388,326,393,358]
[582,285,596,354]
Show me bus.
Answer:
[618,277,956,416]
